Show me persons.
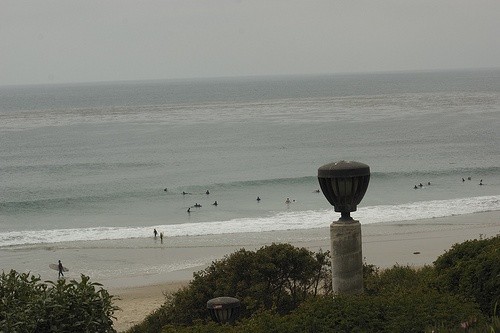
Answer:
[257,196,261,202]
[182,192,186,194]
[205,190,209,194]
[152,228,163,243]
[285,197,295,203]
[213,201,219,205]
[57,260,64,278]
[462,177,483,186]
[164,187,167,192]
[187,203,202,215]
[314,188,320,193]
[413,180,430,189]
